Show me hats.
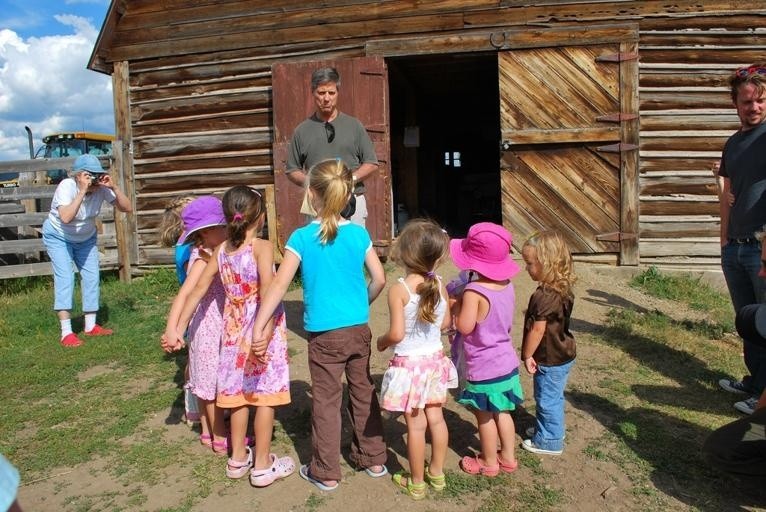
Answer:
[73,154,108,174]
[175,196,227,246]
[449,222,521,281]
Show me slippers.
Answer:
[299,463,338,491]
[365,465,388,478]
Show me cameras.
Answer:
[86,174,101,185]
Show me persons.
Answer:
[252,158,388,492]
[161,196,229,427]
[449,221,526,476]
[520,231,578,457]
[702,226,766,506]
[377,217,459,499]
[165,195,256,454]
[42,152,133,346]
[162,185,296,487]
[283,66,380,230]
[726,185,737,207]
[718,64,766,414]
[446,266,481,375]
[0,451,27,512]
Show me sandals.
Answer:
[424,464,446,491]
[212,435,251,457]
[250,454,296,486]
[200,434,213,447]
[392,470,426,501]
[225,447,254,479]
[462,454,499,477]
[496,449,517,473]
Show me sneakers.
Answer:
[526,427,566,440]
[84,324,113,336]
[61,333,83,347]
[522,439,563,456]
[718,378,747,394]
[734,397,759,415]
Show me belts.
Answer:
[727,237,758,244]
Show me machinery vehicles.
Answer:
[0,126,116,188]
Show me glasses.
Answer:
[736,66,766,78]
